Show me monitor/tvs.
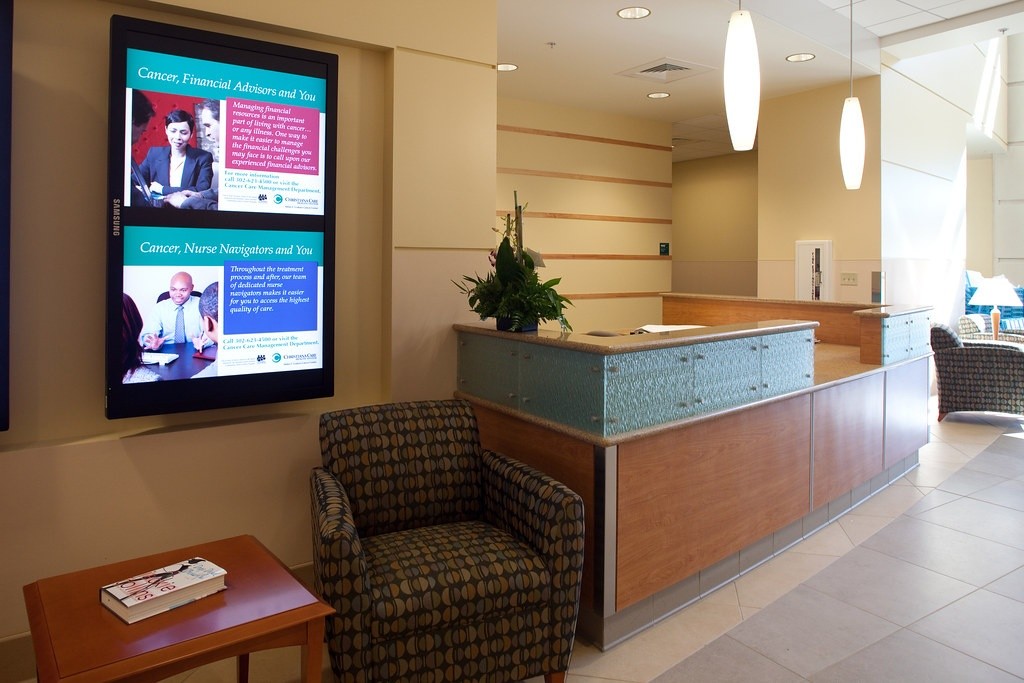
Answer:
[102,13,335,420]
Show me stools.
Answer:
[930,287,1024,421]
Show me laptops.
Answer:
[130,156,175,209]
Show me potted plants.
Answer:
[451,191,573,335]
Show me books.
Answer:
[99,557,228,625]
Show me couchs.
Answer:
[311,399,585,682]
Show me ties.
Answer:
[174,306,186,344]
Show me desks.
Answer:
[23,535,337,683]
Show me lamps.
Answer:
[968,277,1023,340]
[839,0,865,190]
[724,0,760,151]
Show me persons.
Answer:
[123,272,218,383]
[131,89,220,210]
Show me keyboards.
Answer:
[139,352,179,366]
